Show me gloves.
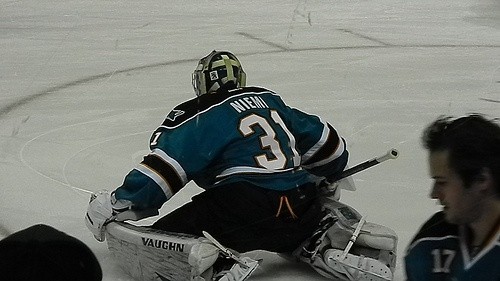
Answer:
[322,182,341,200]
[84,190,125,243]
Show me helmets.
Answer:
[191,49,246,96]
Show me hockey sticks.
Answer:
[322,148,400,185]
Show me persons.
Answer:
[403,113,500,280]
[85,49,397,280]
